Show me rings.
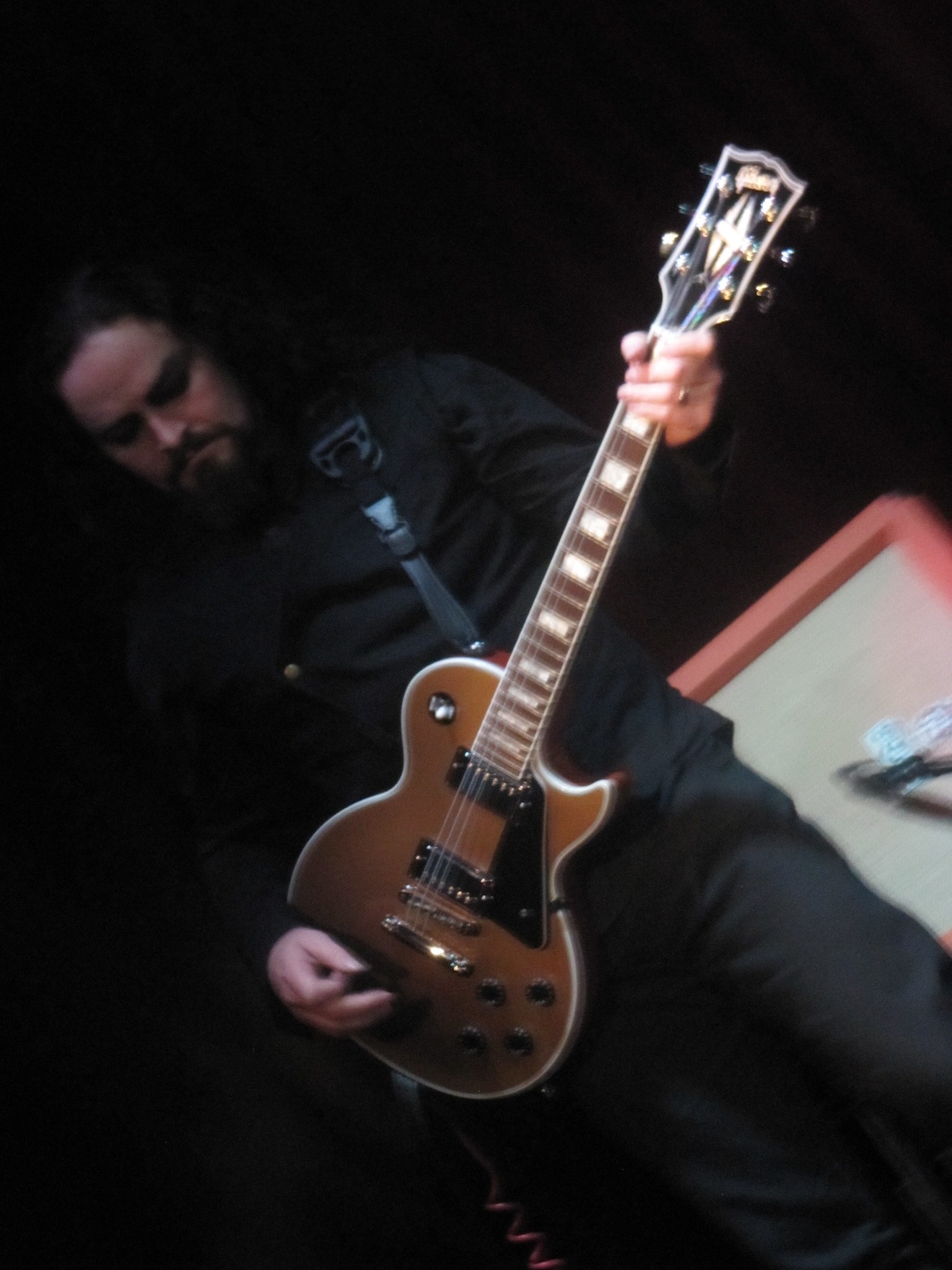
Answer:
[678,386,689,405]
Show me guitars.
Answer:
[279,136,823,1107]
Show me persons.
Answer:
[32,274,952,1270]
